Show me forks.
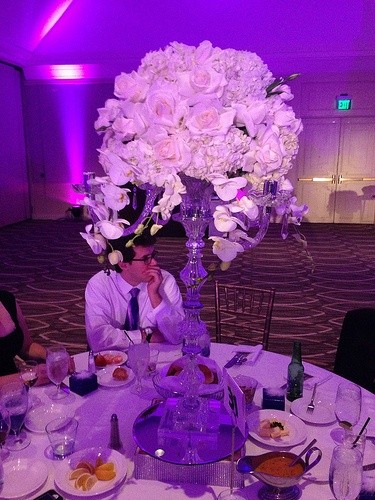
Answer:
[306,383,317,414]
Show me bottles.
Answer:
[87,350,96,373]
[286,342,305,402]
[68,356,75,373]
[109,414,123,450]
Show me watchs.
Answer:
[142,328,153,343]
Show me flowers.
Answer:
[72,37,311,273]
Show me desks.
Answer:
[0,340,375,500]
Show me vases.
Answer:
[120,174,271,436]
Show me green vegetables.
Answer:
[270,422,285,430]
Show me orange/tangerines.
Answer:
[75,455,116,481]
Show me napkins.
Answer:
[283,363,333,389]
[227,344,264,364]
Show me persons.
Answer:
[0,290,76,377]
[84,230,185,356]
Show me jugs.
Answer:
[235,446,323,500]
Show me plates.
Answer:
[348,407,375,437]
[288,444,336,482]
[246,409,307,448]
[94,349,134,387]
[291,397,338,423]
[360,443,375,467]
[0,401,130,500]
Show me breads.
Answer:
[167,362,218,385]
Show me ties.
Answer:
[124,288,140,331]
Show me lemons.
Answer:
[69,468,97,492]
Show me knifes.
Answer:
[224,352,243,369]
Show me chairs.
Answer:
[0,291,24,377]
[214,279,275,350]
[333,307,375,394]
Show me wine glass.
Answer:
[0,381,31,450]
[17,360,39,406]
[0,406,11,461]
[330,382,362,444]
[344,424,367,485]
[129,339,149,394]
[47,345,70,399]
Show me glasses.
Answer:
[123,251,158,264]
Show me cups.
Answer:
[329,446,364,500]
[143,346,158,377]
[0,463,4,495]
[44,417,80,458]
[232,377,258,413]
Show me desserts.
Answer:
[112,368,128,381]
[94,352,123,368]
